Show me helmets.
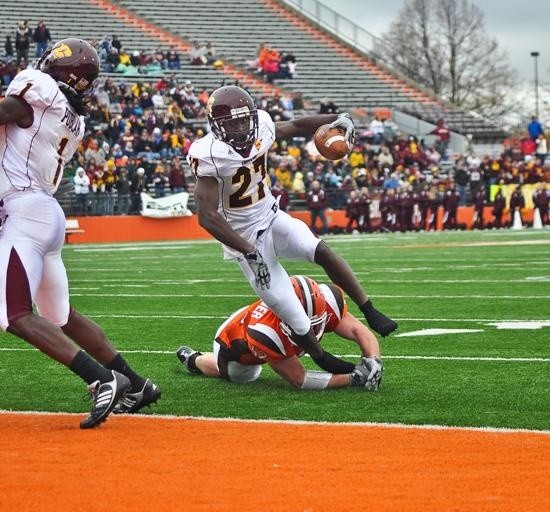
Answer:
[279,275,332,346]
[207,86,261,147]
[37,37,101,94]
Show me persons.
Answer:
[186,84,398,375]
[176,273,385,392]
[0,37,162,429]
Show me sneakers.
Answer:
[176,346,195,373]
[367,310,397,338]
[113,378,161,413]
[319,355,354,373]
[80,370,132,429]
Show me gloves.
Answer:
[353,357,383,392]
[246,247,271,290]
[329,113,357,147]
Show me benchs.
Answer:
[1,0,512,225]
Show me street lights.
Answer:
[531,50,541,118]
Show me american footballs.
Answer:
[313,124,350,160]
